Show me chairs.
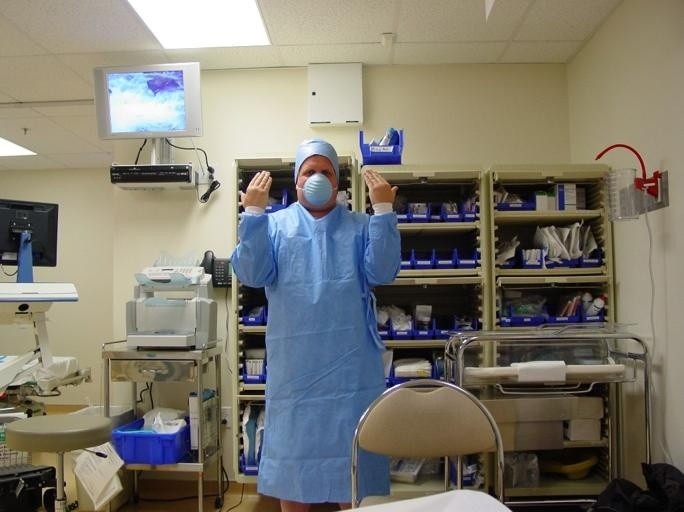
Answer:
[350,379,503,511]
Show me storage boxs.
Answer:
[111,417,190,463]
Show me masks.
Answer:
[295,172,339,206]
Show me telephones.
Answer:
[200,250,232,288]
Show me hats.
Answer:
[295,139,341,184]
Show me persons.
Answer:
[230,140,402,511]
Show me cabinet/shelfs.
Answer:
[233,155,359,485]
[362,165,492,499]
[490,165,648,495]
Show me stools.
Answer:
[5,413,113,512]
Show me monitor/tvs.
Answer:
[0,197,60,267]
[91,61,204,139]
